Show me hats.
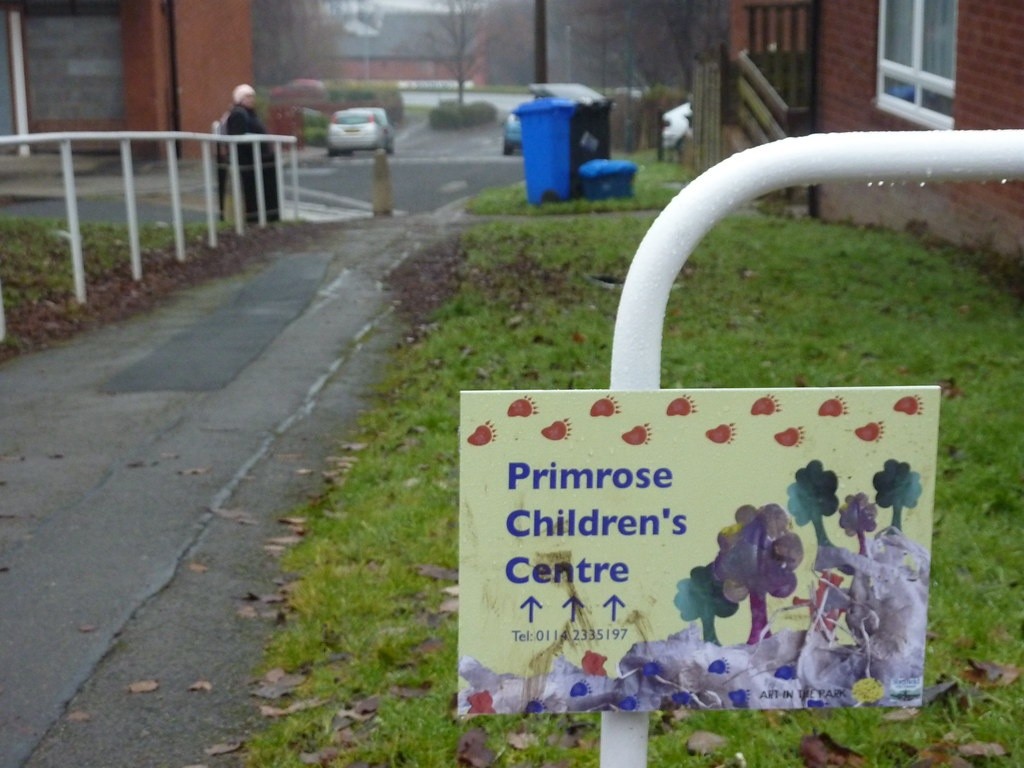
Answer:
[233,84,255,104]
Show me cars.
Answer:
[503,115,523,155]
[720,49,809,123]
[428,98,498,127]
[662,102,693,154]
[326,107,394,156]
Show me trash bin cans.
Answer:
[512,97,576,204]
[529,83,610,198]
[577,160,635,202]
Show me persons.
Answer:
[216,83,279,225]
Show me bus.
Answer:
[269,78,403,123]
